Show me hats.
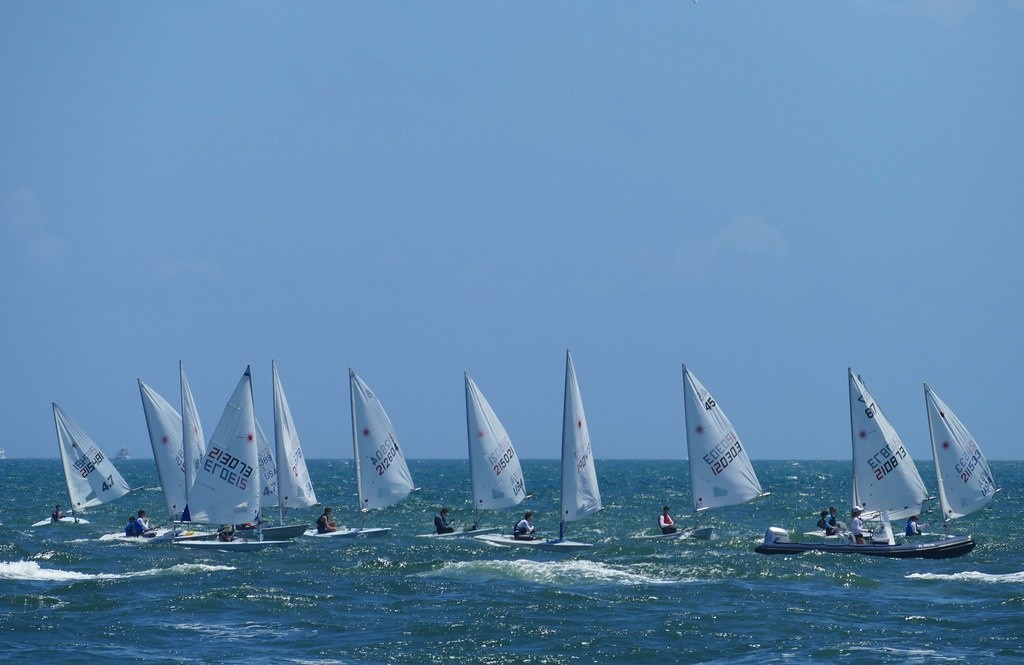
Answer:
[56,505,61,508]
[663,506,671,510]
[829,507,838,513]
[853,506,863,513]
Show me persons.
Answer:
[658,505,677,534]
[906,514,929,536]
[817,506,840,535]
[217,524,237,542]
[316,505,338,534]
[434,507,454,534]
[125,509,149,537]
[51,505,64,522]
[514,511,535,540]
[850,505,880,544]
[242,522,257,530]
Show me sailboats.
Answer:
[416,369,533,540]
[134,359,319,552]
[757,366,1004,559]
[31,402,145,526]
[473,348,607,548]
[630,362,772,541]
[302,366,422,538]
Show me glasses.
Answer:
[444,512,448,515]
